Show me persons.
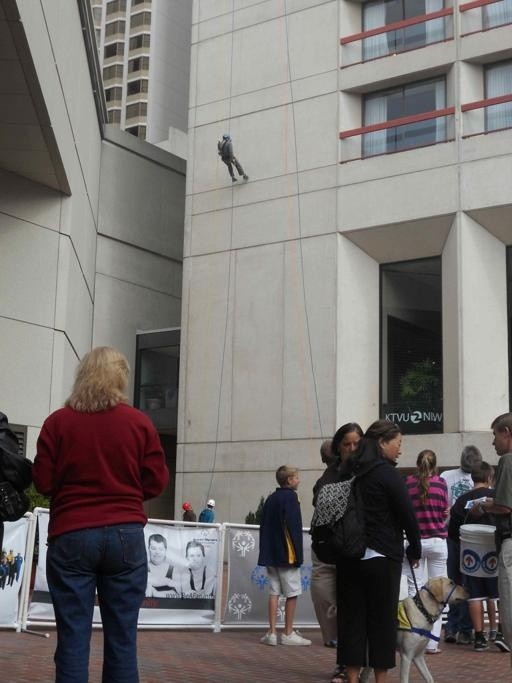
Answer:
[0,411,33,553]
[0,550,23,590]
[197,499,216,523]
[217,133,248,182]
[181,541,216,599]
[145,535,182,598]
[33,346,169,683]
[257,466,312,647]
[183,502,196,528]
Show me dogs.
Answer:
[326,575,471,683]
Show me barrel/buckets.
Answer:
[457,510,499,579]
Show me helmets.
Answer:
[206,499,216,506]
[182,502,190,511]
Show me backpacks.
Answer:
[311,457,385,565]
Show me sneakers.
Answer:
[444,629,511,654]
[259,633,278,646]
[280,631,312,646]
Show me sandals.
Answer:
[331,671,349,682]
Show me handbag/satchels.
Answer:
[0,480,32,522]
